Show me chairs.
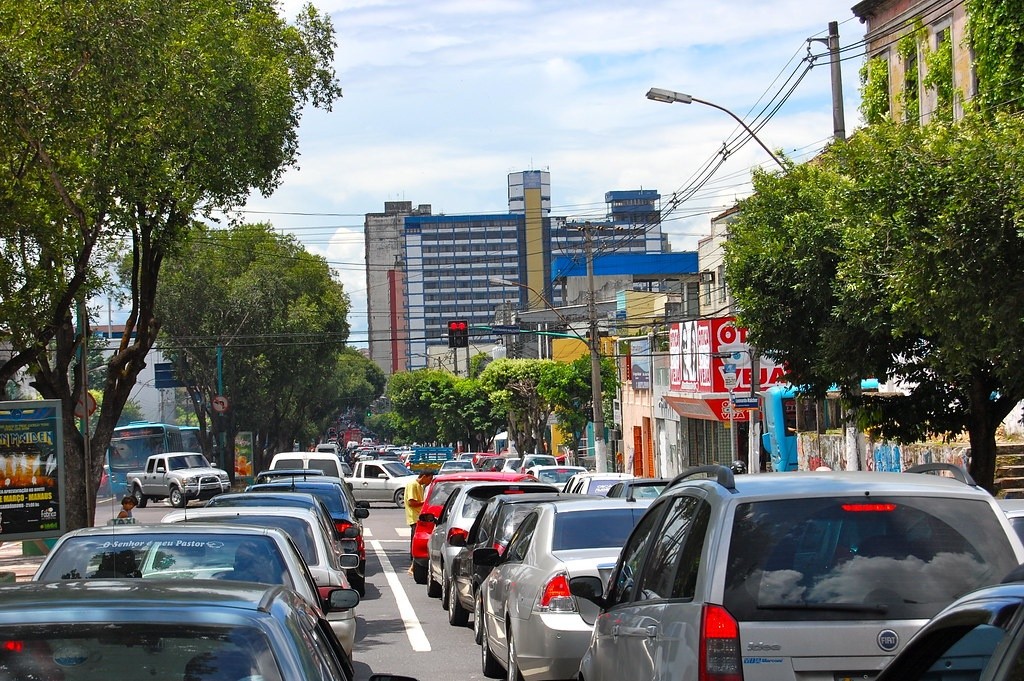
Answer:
[183,651,259,681]
[210,542,283,584]
[82,546,142,578]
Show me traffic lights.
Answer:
[446,320,469,349]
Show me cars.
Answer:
[565,461,1024,681]
[871,579,1024,681]
[0,434,703,681]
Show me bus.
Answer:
[106,421,188,503]
[178,424,205,455]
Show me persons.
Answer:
[404,469,433,578]
[117,496,137,518]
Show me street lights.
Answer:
[487,276,609,474]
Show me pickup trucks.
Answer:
[124,450,234,509]
